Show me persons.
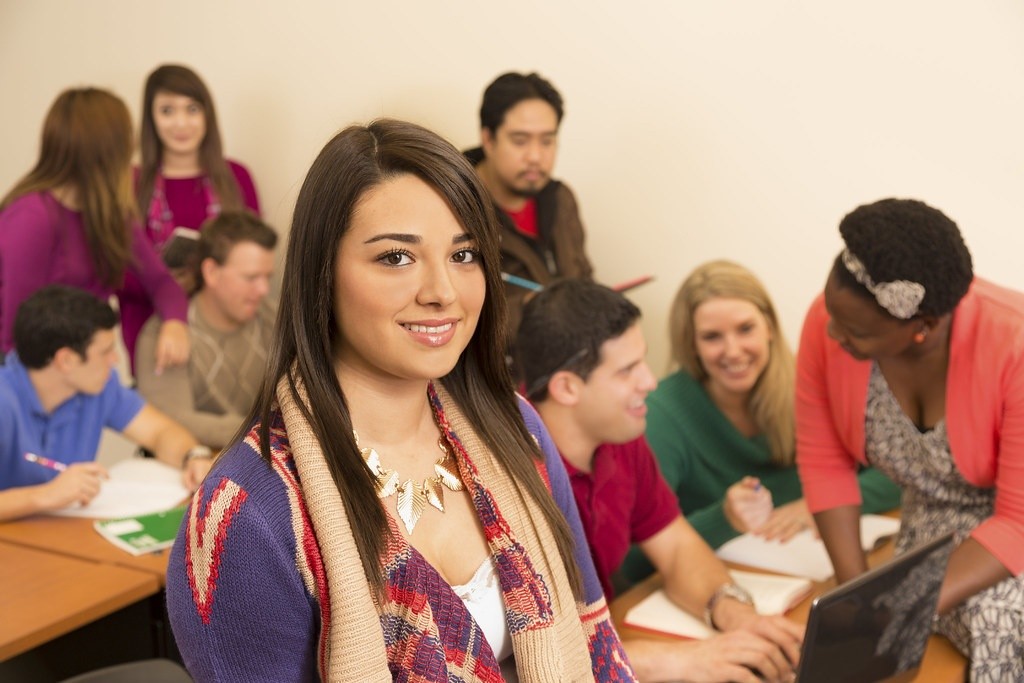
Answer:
[0,87,193,384]
[162,116,638,683]
[616,258,900,595]
[128,209,278,451]
[0,286,217,521]
[131,63,263,282]
[791,197,1023,683]
[455,72,595,395]
[517,277,807,683]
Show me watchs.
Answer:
[180,444,212,468]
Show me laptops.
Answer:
[730,532,957,683]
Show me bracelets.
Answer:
[705,582,754,632]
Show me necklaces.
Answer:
[350,428,467,536]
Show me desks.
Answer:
[0,497,195,589]
[605,510,969,683]
[0,540,161,662]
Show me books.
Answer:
[161,226,201,268]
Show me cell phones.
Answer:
[162,227,201,271]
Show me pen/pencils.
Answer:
[22,451,67,473]
[500,270,547,294]
